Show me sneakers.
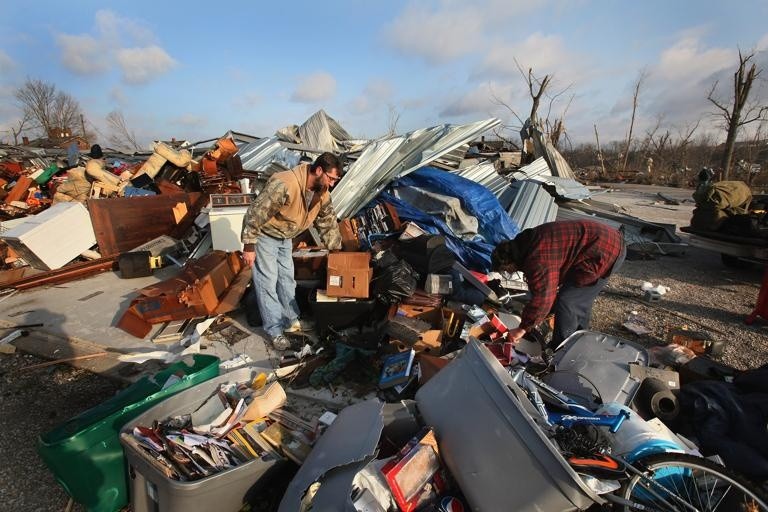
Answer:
[284,319,317,332]
[269,332,291,352]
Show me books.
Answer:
[380,424,455,512]
[377,348,415,388]
[132,384,310,486]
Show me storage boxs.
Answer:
[326,250,372,299]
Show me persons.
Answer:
[491,217,628,350]
[86,143,118,201]
[240,151,344,349]
